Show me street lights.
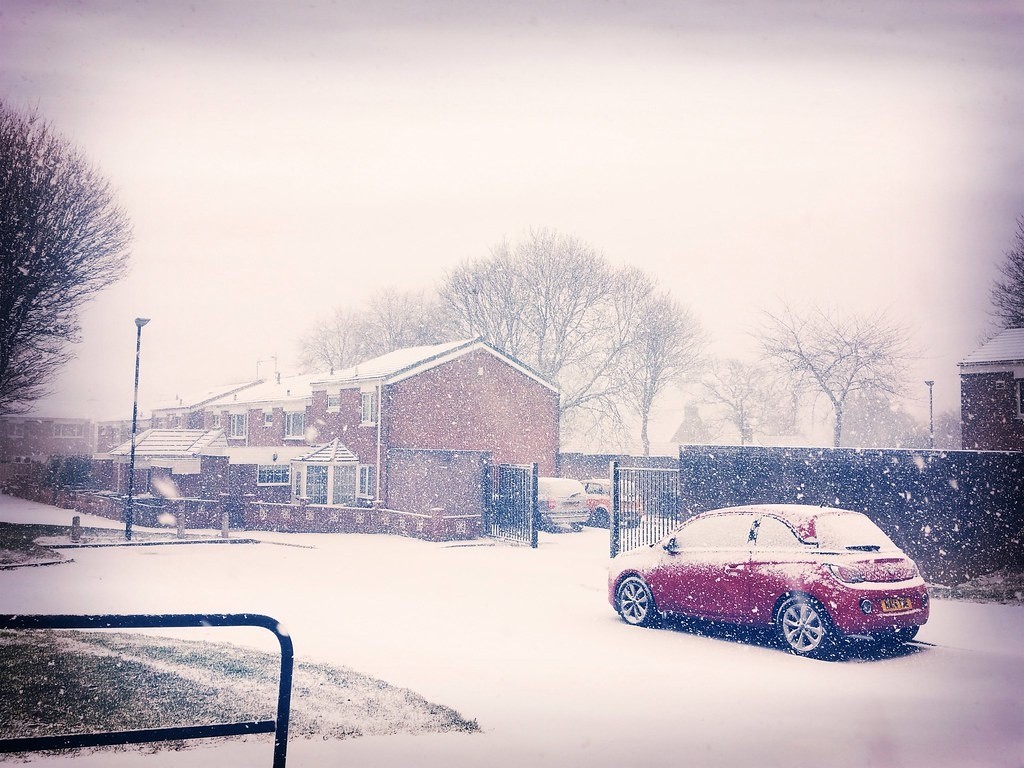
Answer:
[125,316,152,540]
[924,379,935,449]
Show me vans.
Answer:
[490,477,591,533]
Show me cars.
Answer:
[579,479,644,529]
[607,504,930,660]
